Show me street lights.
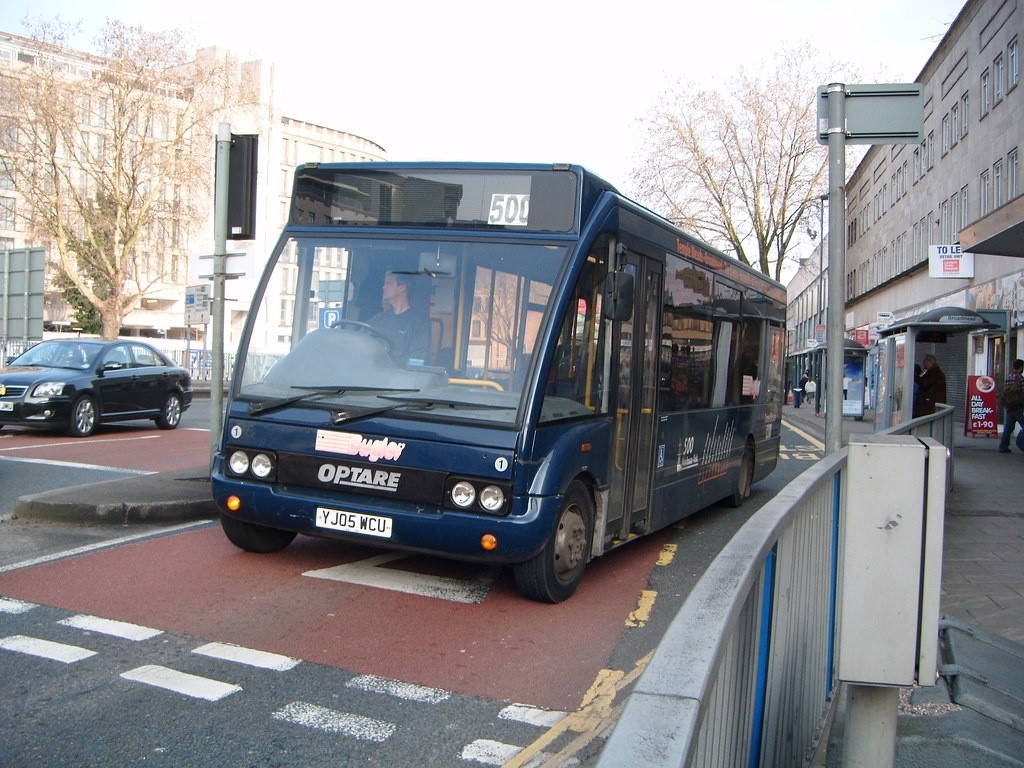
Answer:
[815,194,828,416]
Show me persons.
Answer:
[843,373,852,400]
[998,359,1024,453]
[799,374,808,404]
[359,271,429,367]
[805,378,816,405]
[664,372,699,410]
[921,354,953,413]
[912,364,928,419]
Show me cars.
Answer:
[658,359,671,387]
[0,339,194,434]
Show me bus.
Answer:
[206,161,787,607]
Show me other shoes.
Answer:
[999,448,1011,453]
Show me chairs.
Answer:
[138,354,150,363]
[426,318,654,409]
[110,351,125,363]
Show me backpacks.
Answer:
[914,379,936,418]
[1000,376,1024,407]
